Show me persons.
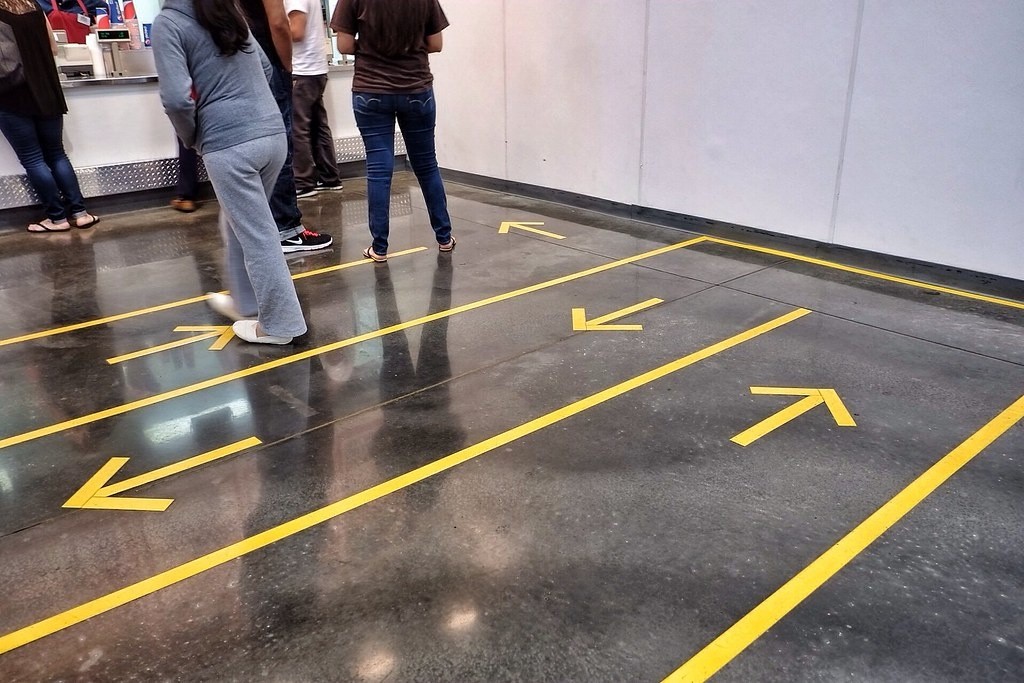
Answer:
[151,0,343,348]
[1,0,100,233]
[330,0,457,263]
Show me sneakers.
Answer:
[313,178,343,190]
[295,186,320,198]
[282,228,334,253]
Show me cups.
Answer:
[96,14,111,28]
[95,7,107,15]
[143,24,152,47]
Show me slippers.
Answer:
[439,236,456,252]
[26,218,72,232]
[363,245,388,262]
[76,213,100,229]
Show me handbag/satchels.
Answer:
[0,20,27,92]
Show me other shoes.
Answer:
[232,320,294,345]
[206,292,261,323]
[170,198,197,213]
